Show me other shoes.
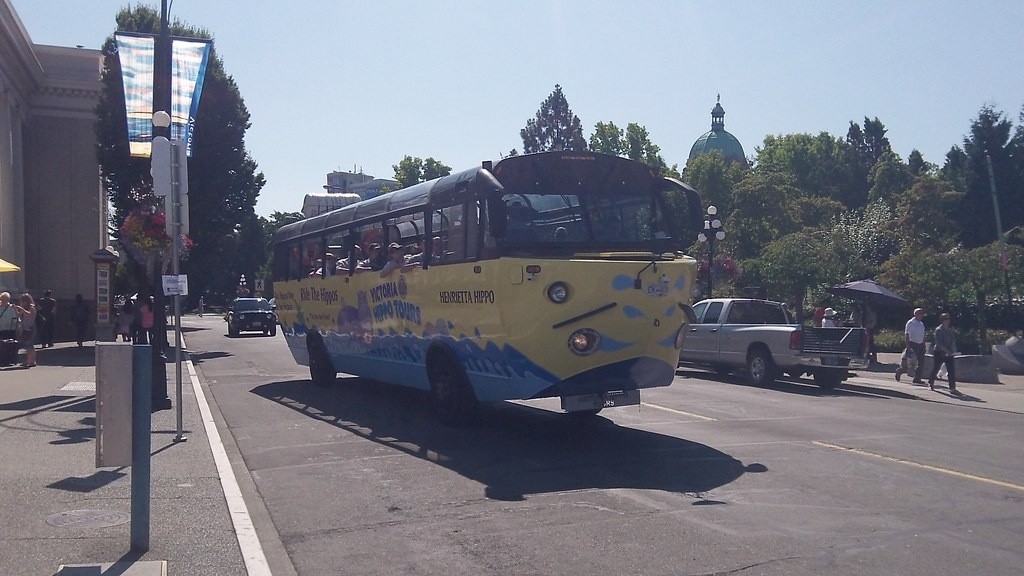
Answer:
[895,369,900,381]
[928,381,934,391]
[48,344,54,347]
[950,389,961,394]
[20,361,36,367]
[913,380,925,384]
[42,344,45,347]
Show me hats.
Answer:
[368,243,381,250]
[388,243,402,249]
[824,308,837,317]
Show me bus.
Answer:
[273,149,705,428]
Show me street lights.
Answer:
[143,108,171,410]
[697,203,726,301]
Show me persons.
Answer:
[37,289,57,348]
[10,293,37,367]
[822,307,838,327]
[308,236,444,277]
[606,215,623,242]
[895,308,926,383]
[813,304,825,326]
[928,312,962,395]
[73,293,89,348]
[0,292,20,367]
[199,296,206,317]
[119,294,154,345]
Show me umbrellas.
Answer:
[826,278,910,303]
[0,257,22,272]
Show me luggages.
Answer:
[0,318,19,366]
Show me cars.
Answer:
[227,297,277,337]
[266,297,280,324]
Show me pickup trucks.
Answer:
[678,297,872,389]
